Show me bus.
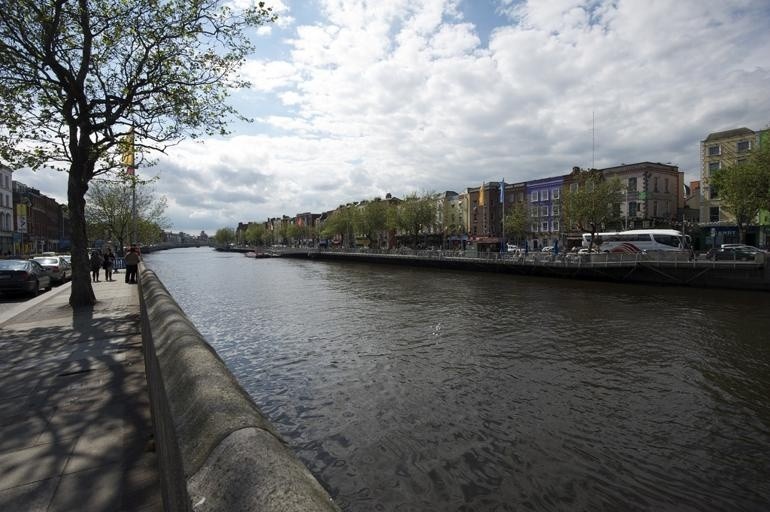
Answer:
[582,228,693,258]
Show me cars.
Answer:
[542,247,555,252]
[0,259,52,297]
[33,255,73,285]
[508,245,526,252]
[578,249,607,255]
[706,244,768,261]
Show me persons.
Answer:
[88,243,140,285]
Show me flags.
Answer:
[478,182,485,207]
[126,166,134,175]
[497,178,505,203]
[121,124,134,165]
